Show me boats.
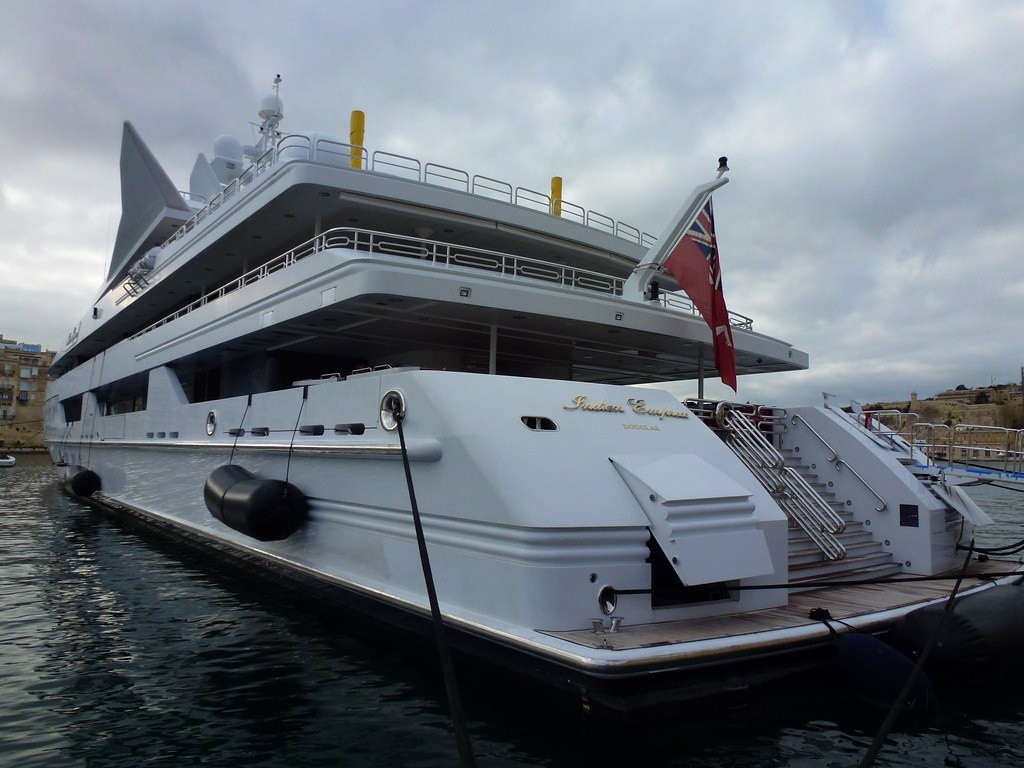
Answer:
[0,455,17,468]
[39,72,1023,670]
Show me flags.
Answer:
[658,180,740,396]
